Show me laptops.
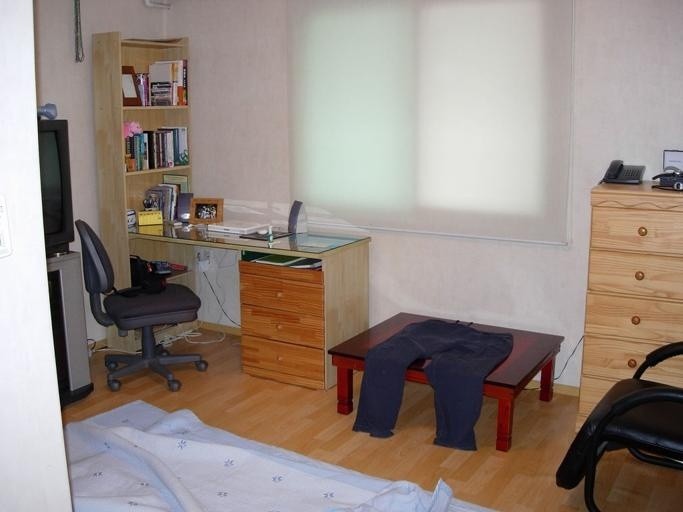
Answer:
[208,221,269,234]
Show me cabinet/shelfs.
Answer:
[90,33,195,353]
[575,179,683,432]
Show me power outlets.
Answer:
[127,225,370,389]
[196,248,213,274]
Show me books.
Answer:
[242,253,270,262]
[255,254,305,266]
[289,259,322,268]
[117,53,194,229]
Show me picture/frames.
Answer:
[119,66,142,106]
[191,198,224,225]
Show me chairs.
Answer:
[76,220,208,391]
[556,338,683,512]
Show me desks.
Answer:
[46,249,93,405]
[327,312,563,451]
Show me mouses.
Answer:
[257,230,268,234]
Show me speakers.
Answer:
[288,201,308,234]
[178,193,194,224]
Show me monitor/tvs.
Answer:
[38,120,74,258]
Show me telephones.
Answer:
[605,160,645,183]
